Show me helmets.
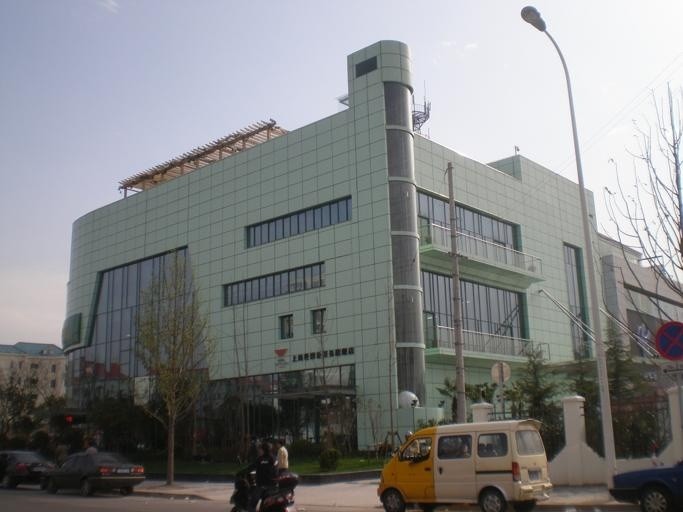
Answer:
[236,480,250,496]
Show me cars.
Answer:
[610,461,683,511]
[0,450,54,489]
[46,451,147,497]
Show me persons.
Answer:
[235,442,279,512]
[273,440,288,475]
[85,442,98,453]
[405,431,419,457]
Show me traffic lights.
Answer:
[61,414,84,425]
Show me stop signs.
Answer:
[657,321,682,360]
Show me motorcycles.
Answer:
[229,469,298,512]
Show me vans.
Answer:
[378,419,553,511]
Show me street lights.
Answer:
[521,5,616,469]
